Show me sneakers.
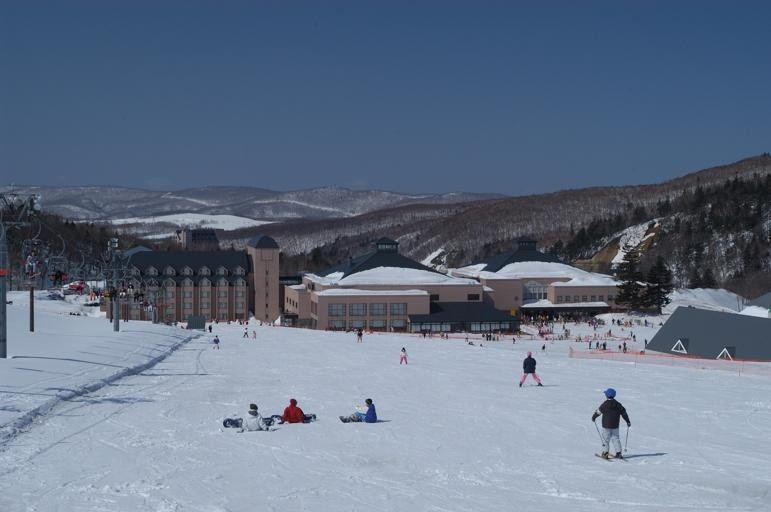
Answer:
[602,452,620,459]
[519,384,522,386]
[538,383,542,386]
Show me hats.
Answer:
[604,388,615,398]
[291,400,297,405]
[527,352,532,356]
[250,404,258,411]
[365,398,372,405]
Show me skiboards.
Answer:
[595,453,628,462]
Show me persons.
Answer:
[208,318,257,350]
[25,250,154,313]
[592,389,631,459]
[519,352,542,387]
[238,403,269,433]
[356,311,648,363]
[339,399,377,424]
[279,399,312,425]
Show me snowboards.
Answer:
[339,414,364,423]
[271,414,316,424]
[223,419,274,428]
